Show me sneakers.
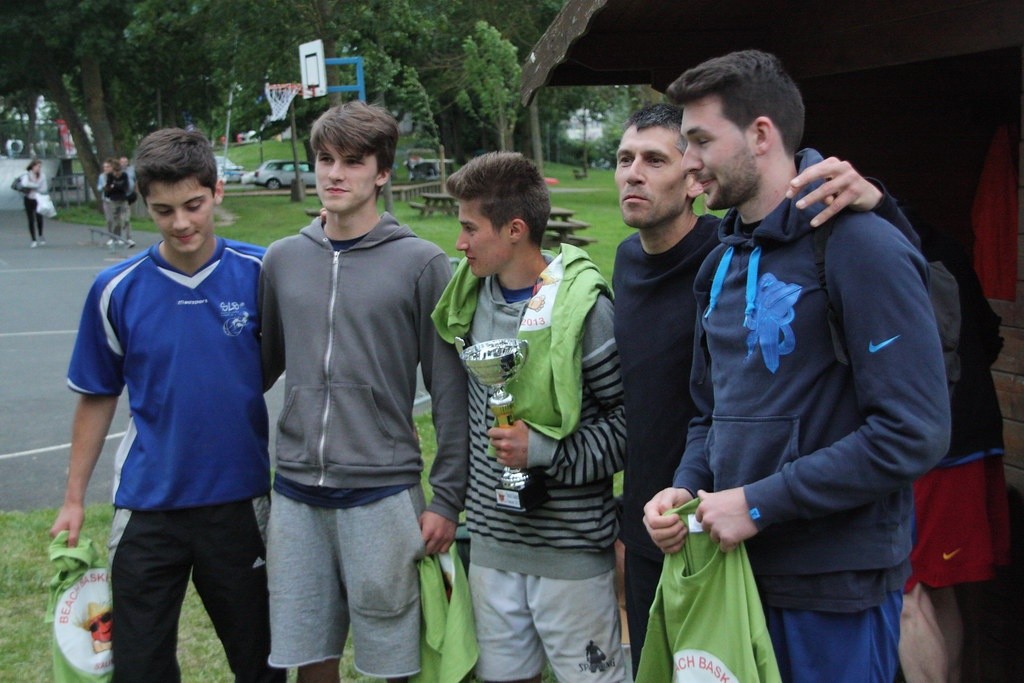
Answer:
[40,241,45,246]
[106,238,114,245]
[127,240,135,248]
[118,240,124,245]
[31,241,37,247]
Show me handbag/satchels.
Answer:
[127,190,137,205]
[10,174,32,193]
[35,192,57,218]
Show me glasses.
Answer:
[89,611,111,632]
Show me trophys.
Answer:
[453,333,550,512]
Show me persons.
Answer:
[96,156,136,248]
[263,51,1008,683]
[19,160,50,247]
[49,128,269,683]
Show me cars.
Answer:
[213,156,244,185]
[240,159,316,190]
[389,159,455,182]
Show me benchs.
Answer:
[568,233,598,244]
[408,201,425,209]
[449,202,459,207]
[568,217,589,229]
[547,221,572,229]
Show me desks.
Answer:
[421,193,459,217]
[550,206,577,243]
